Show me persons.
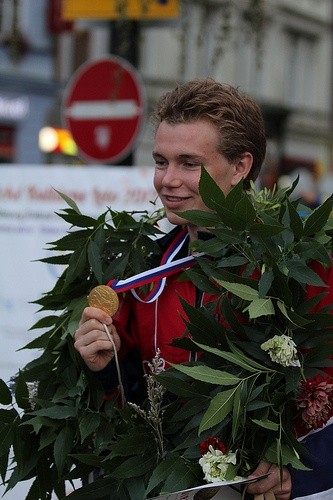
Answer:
[58,77,332,500]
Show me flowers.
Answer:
[198,446,246,485]
[260,331,302,370]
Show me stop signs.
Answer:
[60,55,149,165]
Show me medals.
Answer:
[86,284,120,317]
